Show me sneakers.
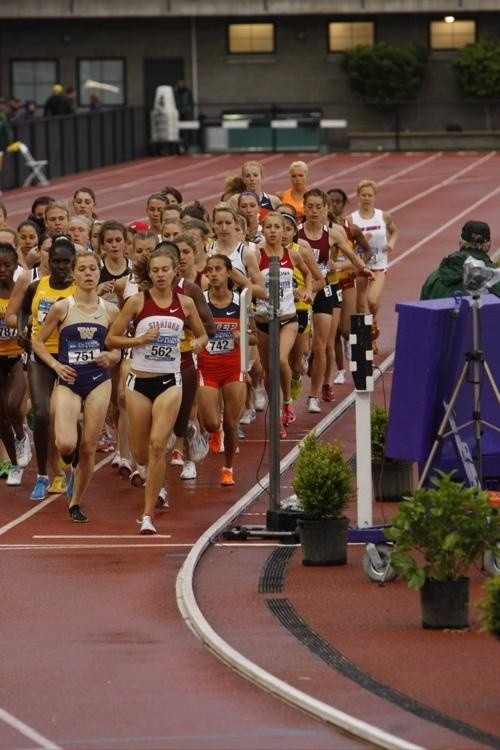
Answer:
[220,467,236,485]
[0,459,12,478]
[69,504,88,523]
[185,423,247,456]
[14,432,32,466]
[96,432,168,508]
[49,473,67,492]
[30,474,50,500]
[179,460,197,480]
[168,448,183,465]
[140,516,157,534]
[239,369,348,438]
[6,465,24,485]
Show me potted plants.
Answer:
[291,428,360,565]
[370,403,419,501]
[383,467,493,630]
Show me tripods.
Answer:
[413,315,500,493]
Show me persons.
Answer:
[18,100,37,121]
[0,96,8,123]
[0,161,399,534]
[420,219,499,302]
[89,94,101,110]
[174,78,196,155]
[66,86,76,106]
[44,83,72,115]
[8,96,19,121]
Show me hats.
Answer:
[461,220,490,244]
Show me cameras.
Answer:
[464,253,488,293]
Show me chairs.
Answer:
[20,144,49,187]
